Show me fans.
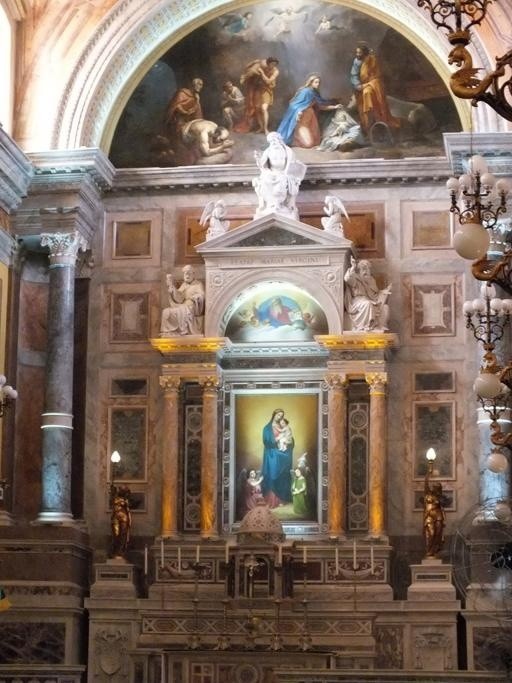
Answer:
[447,494,512,623]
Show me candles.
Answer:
[335,542,375,575]
[143,541,202,577]
[278,545,308,564]
[224,543,230,564]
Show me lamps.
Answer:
[0,374,19,417]
[444,150,512,476]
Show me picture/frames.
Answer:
[410,281,457,339]
[106,374,150,514]
[410,209,455,251]
[111,217,155,260]
[413,368,457,512]
[107,288,153,345]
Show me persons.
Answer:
[239,297,319,331]
[199,200,230,240]
[344,255,393,334]
[110,487,131,559]
[249,130,307,221]
[422,465,452,558]
[321,196,350,238]
[160,263,205,337]
[237,410,316,522]
[156,11,401,165]
